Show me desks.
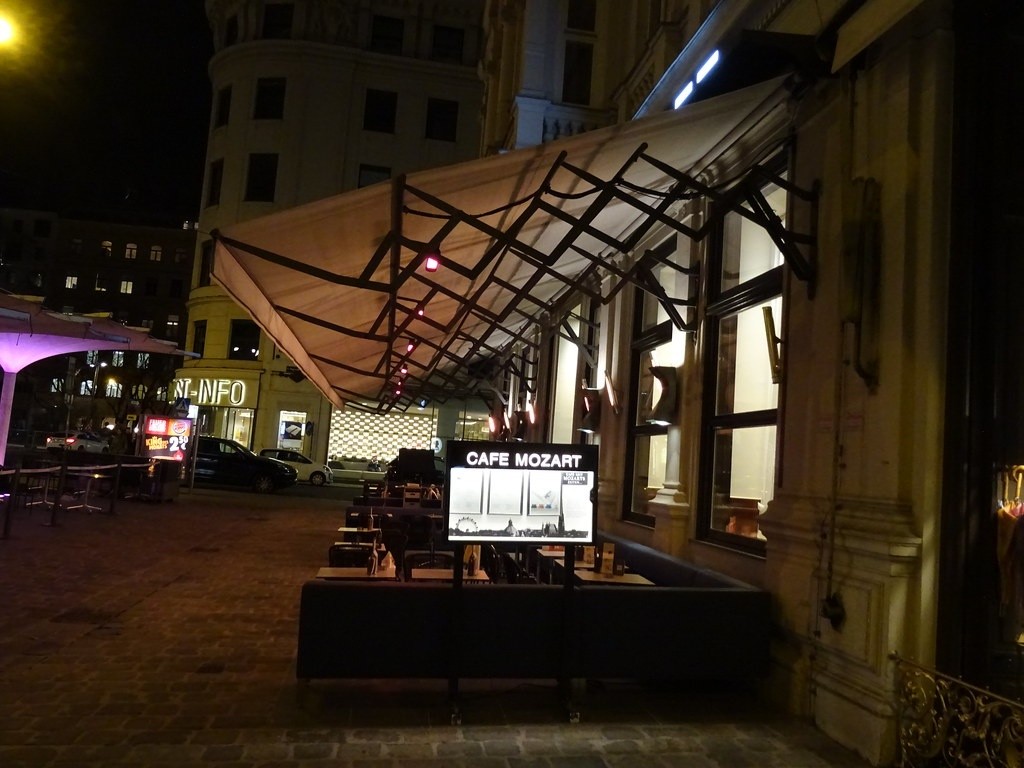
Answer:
[412,568,492,584]
[536,546,601,561]
[314,563,397,580]
[333,541,386,555]
[553,559,631,573]
[337,527,381,535]
[576,568,657,587]
[0,450,182,539]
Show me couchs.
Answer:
[341,462,386,471]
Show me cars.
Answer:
[45,430,110,454]
[258,447,334,486]
[188,436,298,493]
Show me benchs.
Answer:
[296,498,764,703]
[331,469,385,480]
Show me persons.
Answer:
[419,475,440,503]
[367,456,382,472]
[101,419,132,455]
[46,393,68,432]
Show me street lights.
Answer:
[63,360,108,438]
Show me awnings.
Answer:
[209,70,818,412]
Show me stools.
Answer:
[122,455,153,502]
[23,452,65,511]
[66,452,104,515]
